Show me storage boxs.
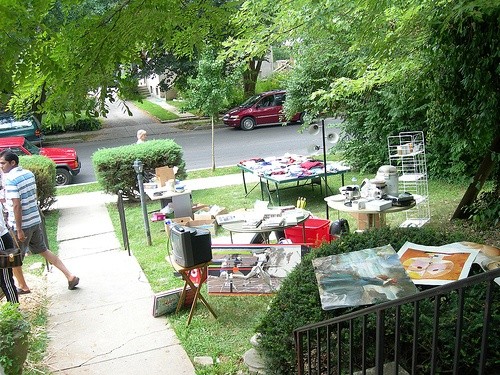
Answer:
[365,201,392,212]
[153,203,227,237]
[151,165,179,187]
[285,219,330,248]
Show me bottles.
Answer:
[342,187,354,201]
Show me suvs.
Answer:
[0,136,81,186]
[0,112,44,148]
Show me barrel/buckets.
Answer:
[377,165,399,197]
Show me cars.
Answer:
[221,89,306,132]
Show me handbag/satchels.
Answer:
[0,248,23,268]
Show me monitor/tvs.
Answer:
[171,225,212,267]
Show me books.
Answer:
[215,200,305,229]
[144,177,185,197]
[365,199,393,211]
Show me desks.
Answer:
[327,194,416,231]
[144,189,192,218]
[238,154,351,207]
[223,205,310,244]
[166,256,218,325]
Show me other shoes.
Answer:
[16,287,30,294]
[68,276,80,290]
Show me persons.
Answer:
[0,149,80,305]
[137,129,146,143]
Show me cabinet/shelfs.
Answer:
[387,131,431,231]
